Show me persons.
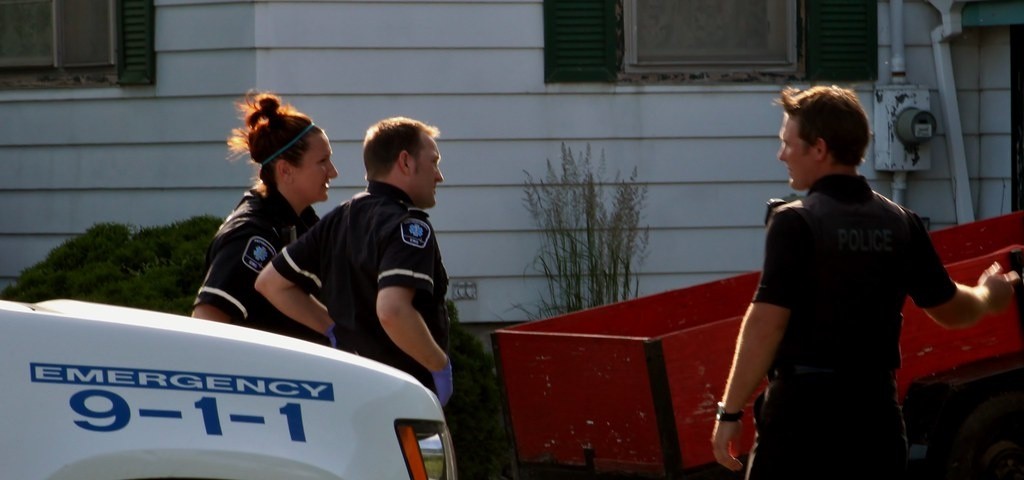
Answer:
[711,84,1021,480]
[254,116,455,412]
[191,94,337,347]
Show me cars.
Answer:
[0,297,456,479]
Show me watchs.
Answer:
[714,402,746,423]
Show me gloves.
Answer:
[425,360,457,405]
[326,329,340,351]
